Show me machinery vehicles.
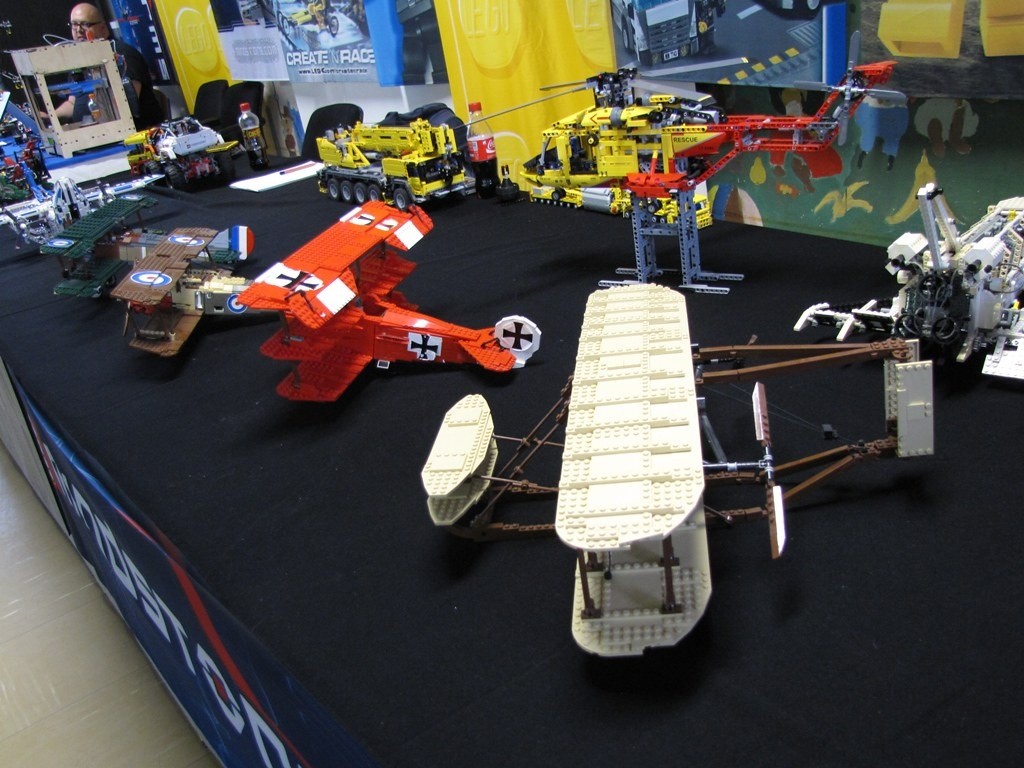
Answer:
[276,0,341,39]
[317,119,476,211]
[125,117,242,189]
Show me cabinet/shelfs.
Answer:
[10,37,139,160]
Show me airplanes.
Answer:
[104,219,276,360]
[234,194,543,407]
[1,160,164,247]
[787,179,1021,392]
[419,281,940,664]
[41,189,169,307]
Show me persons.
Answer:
[35,4,161,134]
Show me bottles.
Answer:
[237,102,270,172]
[467,102,500,199]
[88,93,101,122]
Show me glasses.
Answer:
[67,21,104,29]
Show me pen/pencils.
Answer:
[280,162,317,175]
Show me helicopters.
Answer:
[473,48,910,299]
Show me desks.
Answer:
[0,159,1023,767]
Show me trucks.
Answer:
[612,0,725,64]
